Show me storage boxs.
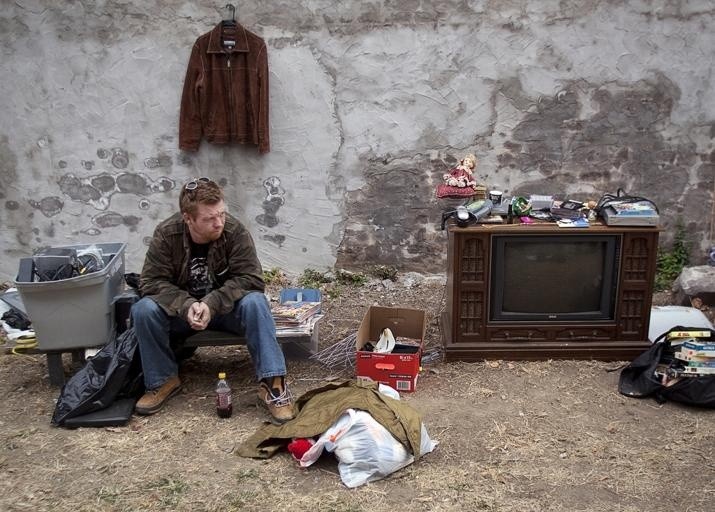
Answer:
[13,241,127,348]
[354,305,426,393]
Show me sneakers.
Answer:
[136,376,184,415]
[257,376,296,424]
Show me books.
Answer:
[269,300,325,332]
[654,330,715,386]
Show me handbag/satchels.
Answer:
[618,326,715,408]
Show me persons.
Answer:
[130,177,296,422]
[436,153,476,208]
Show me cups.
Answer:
[489,191,502,207]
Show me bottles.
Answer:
[216,373,232,418]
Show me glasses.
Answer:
[185,176,210,190]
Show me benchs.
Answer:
[4,315,324,387]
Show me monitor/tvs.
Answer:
[492,236,616,322]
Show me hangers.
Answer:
[220,2,238,28]
